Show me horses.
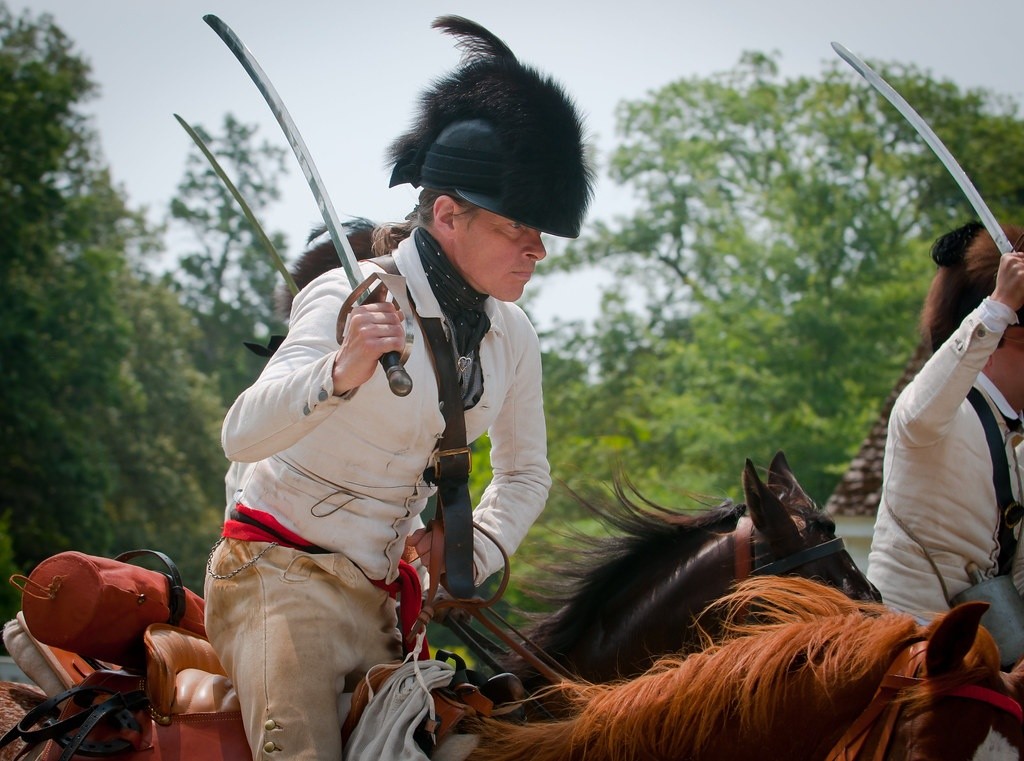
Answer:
[1,448,1024,761]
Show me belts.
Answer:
[368,252,479,603]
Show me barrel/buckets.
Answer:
[950,575,1024,667]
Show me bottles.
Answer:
[964,562,984,585]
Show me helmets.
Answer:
[389,15,592,236]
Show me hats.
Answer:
[276,216,387,319]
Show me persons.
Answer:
[865,223,1024,626]
[204,120,583,761]
